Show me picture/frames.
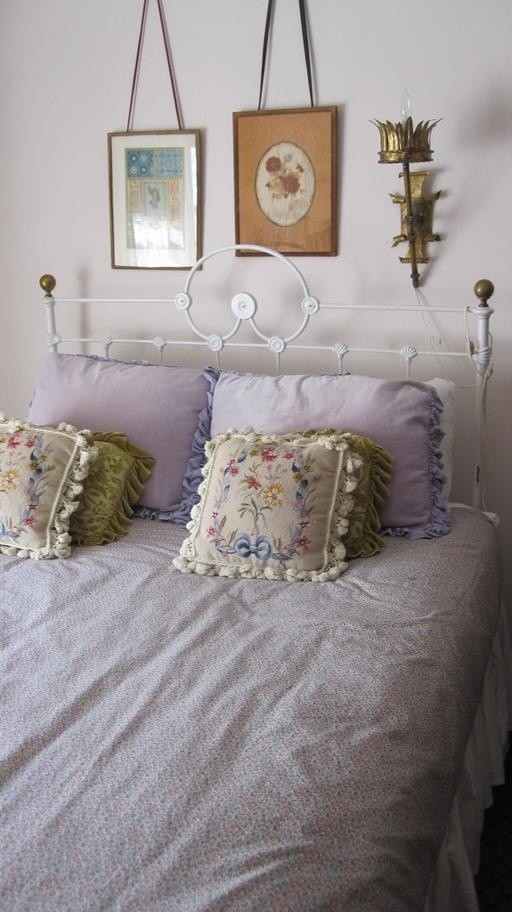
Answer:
[108,103,340,272]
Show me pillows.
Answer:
[171,426,363,582]
[173,364,452,540]
[293,428,396,560]
[421,376,457,512]
[25,353,210,523]
[2,416,99,560]
[68,431,157,546]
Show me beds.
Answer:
[0,242,500,912]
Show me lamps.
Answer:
[370,87,449,290]
